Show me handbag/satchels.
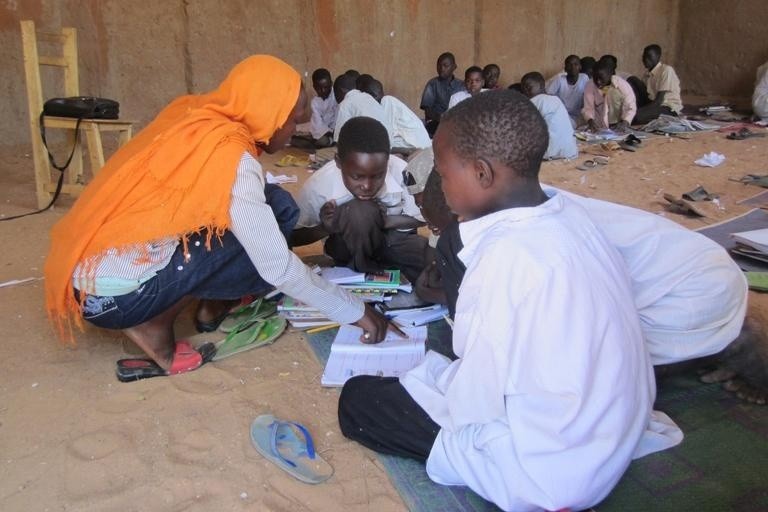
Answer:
[43,95,118,119]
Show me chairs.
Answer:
[18,13,139,214]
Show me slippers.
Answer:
[576,133,641,171]
[116,342,215,382]
[727,126,764,140]
[663,186,719,217]
[250,413,333,484]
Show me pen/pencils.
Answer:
[306,323,340,334]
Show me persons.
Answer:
[309,68,431,161]
[337,89,658,510]
[42,54,388,383]
[546,44,682,134]
[421,161,750,383]
[420,52,577,160]
[288,115,438,274]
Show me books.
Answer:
[729,227,768,263]
[262,259,451,386]
[574,126,618,142]
